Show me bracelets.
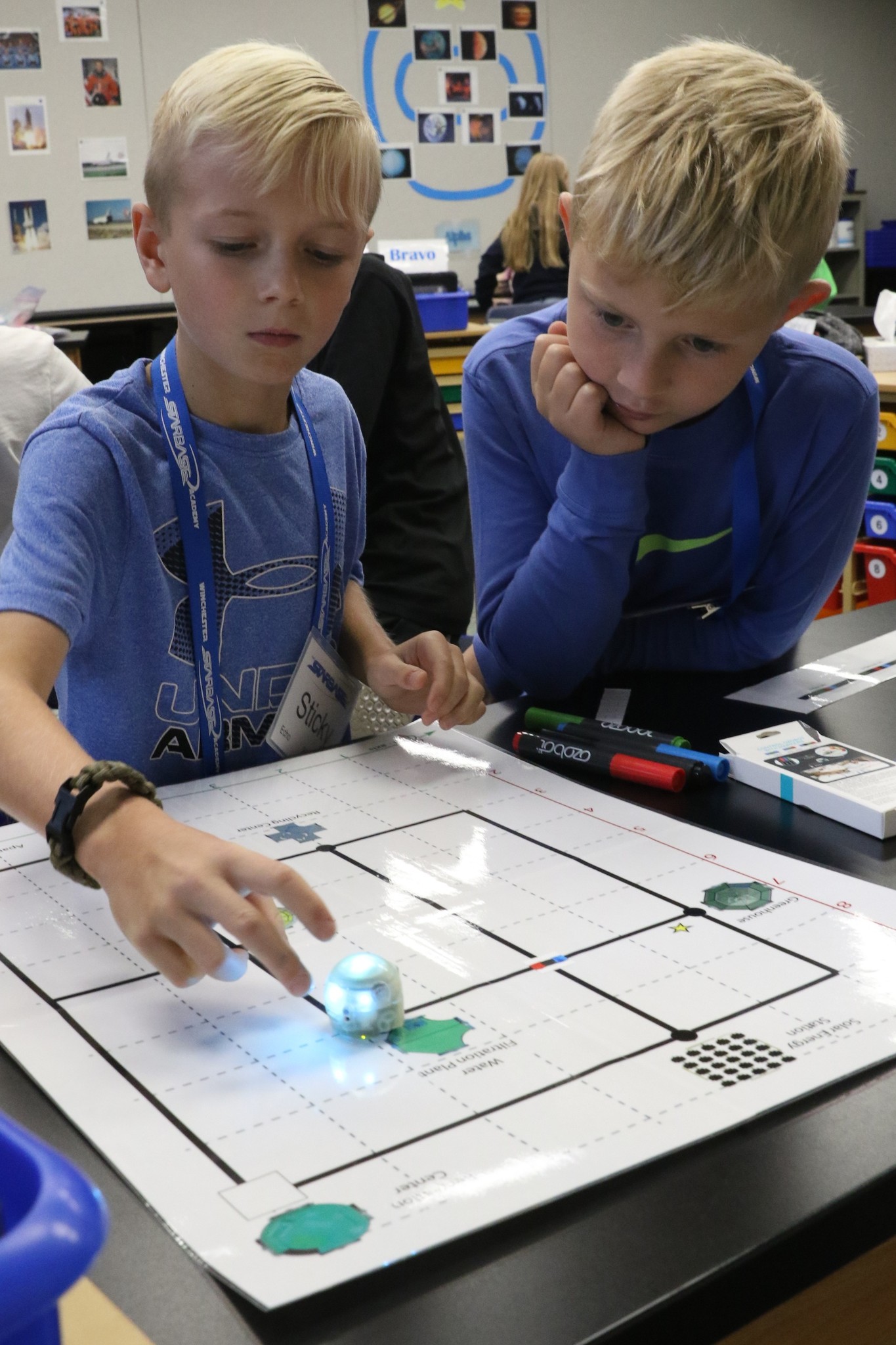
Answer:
[43,758,162,888]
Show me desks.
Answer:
[0,601,896,1345]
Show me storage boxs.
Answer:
[863,335,896,373]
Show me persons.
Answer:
[460,37,879,699]
[0,322,92,557]
[474,152,569,313]
[0,40,487,998]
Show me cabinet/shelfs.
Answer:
[825,190,876,334]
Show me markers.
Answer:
[514,706,732,793]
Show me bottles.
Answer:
[836,216,857,247]
[846,168,858,191]
